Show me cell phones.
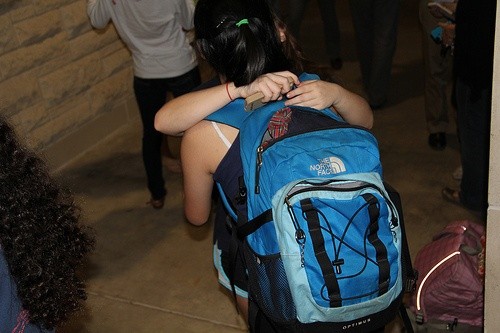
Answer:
[244,76,294,113]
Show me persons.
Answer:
[418,0,497,209]
[181,0,410,333]
[285,0,342,74]
[154,8,374,137]
[0,118,96,333]
[86,0,203,210]
[349,0,400,110]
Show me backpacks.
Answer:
[205,72,420,333]
[412,218,486,326]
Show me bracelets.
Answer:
[225,82,233,102]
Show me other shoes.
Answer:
[367,97,384,110]
[150,197,166,210]
[427,131,447,150]
[440,187,461,205]
[329,57,343,70]
